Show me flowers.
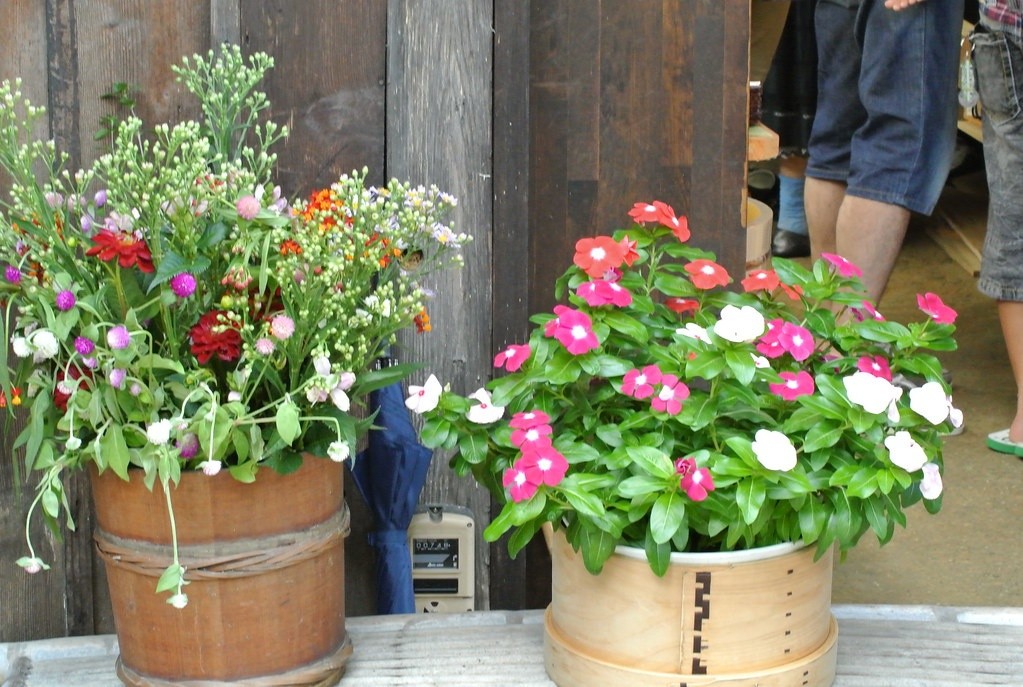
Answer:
[401,200,963,580]
[0,42,474,609]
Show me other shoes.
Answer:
[987,429,1022,458]
[771,231,810,257]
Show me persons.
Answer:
[969,1,1023,455]
[760,3,819,257]
[802,0,963,434]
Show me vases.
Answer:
[82,437,355,687]
[540,514,839,685]
